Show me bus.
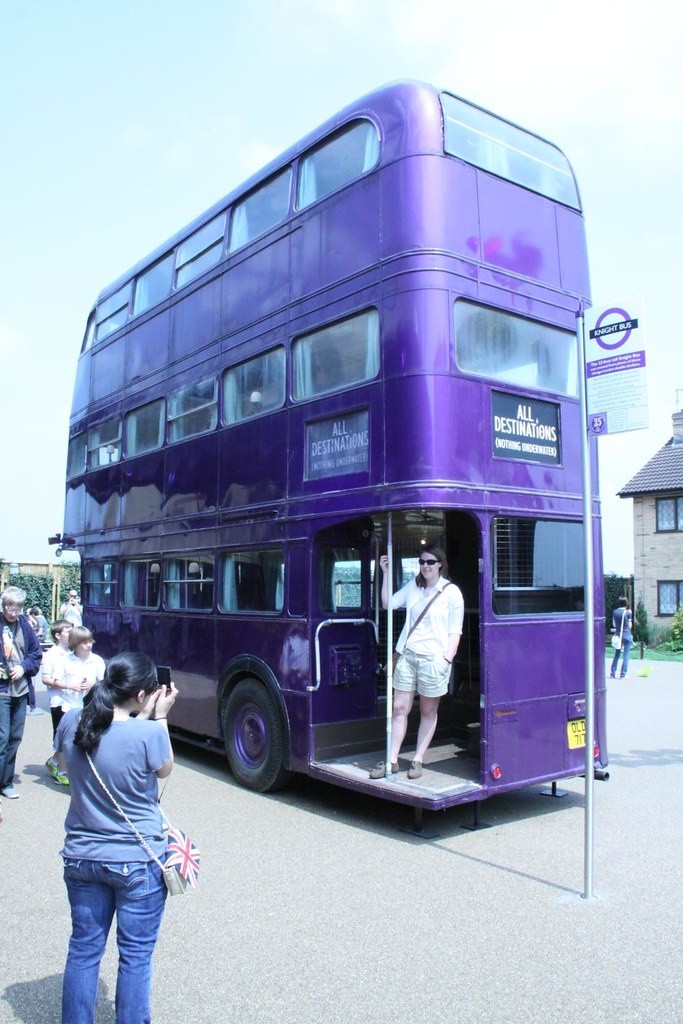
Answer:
[49,79,610,816]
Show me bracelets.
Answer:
[444,658,450,664]
[155,717,168,721]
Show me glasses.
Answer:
[419,558,440,565]
[2,602,23,616]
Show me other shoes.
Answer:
[369,761,399,779]
[611,675,619,679]
[2,787,18,798]
[621,676,630,680]
[408,761,422,779]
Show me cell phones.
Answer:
[156,666,171,691]
[73,595,77,599]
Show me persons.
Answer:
[60,590,83,627]
[51,626,106,714]
[610,595,633,681]
[25,606,48,643]
[370,547,464,779]
[0,586,42,799]
[52,650,178,1023]
[41,621,72,786]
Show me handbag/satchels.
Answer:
[162,825,201,896]
[611,635,622,649]
[381,646,401,674]
[0,665,10,683]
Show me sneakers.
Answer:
[45,758,69,785]
